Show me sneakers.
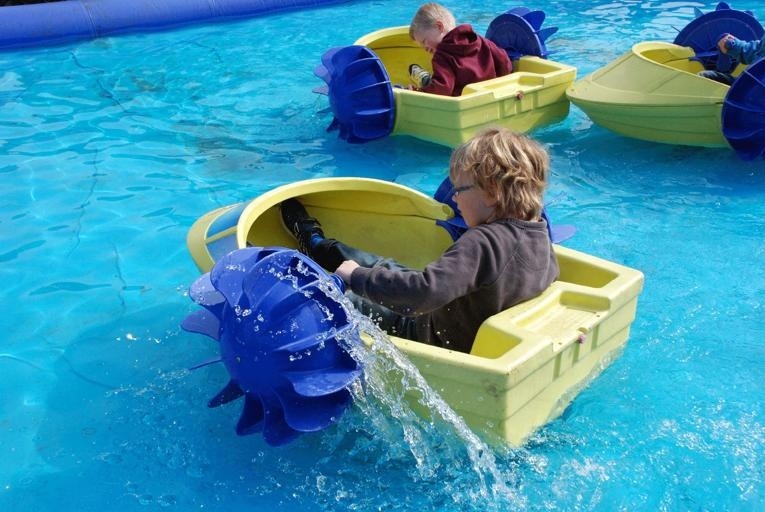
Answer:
[280,199,325,260]
[409,63,430,88]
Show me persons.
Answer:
[279,126,560,354]
[394,2,511,96]
[697,31,765,82]
